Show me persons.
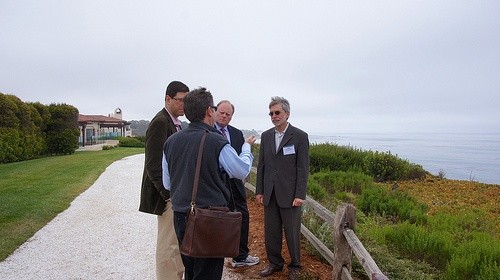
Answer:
[213,100,259,268]
[138,81,189,280]
[255,97,309,280]
[162,87,256,280]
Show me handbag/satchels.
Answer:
[180,208,242,258]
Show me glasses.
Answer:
[269,111,282,116]
[211,106,217,111]
[172,96,182,102]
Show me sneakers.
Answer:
[232,256,259,266]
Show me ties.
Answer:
[220,128,227,139]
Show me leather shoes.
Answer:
[259,266,283,276]
